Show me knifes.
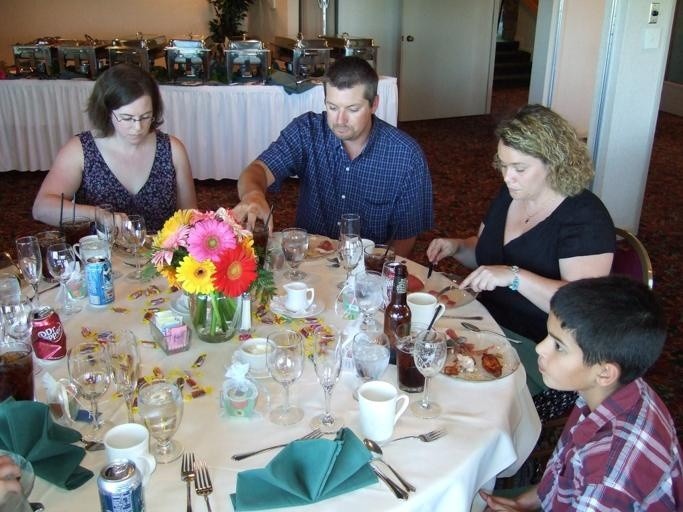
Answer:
[426,259,434,280]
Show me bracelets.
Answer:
[509,265,520,291]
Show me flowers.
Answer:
[142,206,281,339]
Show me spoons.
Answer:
[460,321,522,345]
[361,439,416,493]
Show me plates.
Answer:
[411,271,479,309]
[306,234,336,259]
[231,352,274,380]
[168,292,190,317]
[434,329,520,384]
[269,296,325,320]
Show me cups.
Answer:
[392,321,437,393]
[104,423,156,490]
[406,291,445,337]
[59,215,92,250]
[282,281,314,313]
[238,222,268,269]
[362,243,395,275]
[339,213,361,239]
[357,381,411,446]
[71,234,109,266]
[242,337,276,374]
[0,340,33,402]
[35,230,69,283]
[360,239,374,255]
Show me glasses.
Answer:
[111,111,155,127]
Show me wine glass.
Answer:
[45,241,81,315]
[93,204,122,281]
[136,379,185,465]
[352,330,390,402]
[120,214,152,282]
[281,228,307,281]
[335,232,364,290]
[354,269,386,332]
[265,330,305,427]
[105,327,140,423]
[14,235,45,309]
[310,324,344,434]
[411,330,448,420]
[65,341,112,440]
[0,270,25,344]
[0,294,42,375]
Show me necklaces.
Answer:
[523,196,555,225]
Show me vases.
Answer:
[186,290,245,343]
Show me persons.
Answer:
[31,64,199,243]
[425,102,617,398]
[228,56,435,260]
[477,275,683,510]
[0,454,21,494]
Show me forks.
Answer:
[436,286,472,296]
[377,428,448,448]
[179,452,195,512]
[232,427,324,463]
[194,460,213,512]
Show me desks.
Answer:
[0,75,400,180]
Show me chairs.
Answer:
[515,223,655,456]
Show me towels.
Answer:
[228,424,380,510]
[2,393,96,491]
[497,324,552,397]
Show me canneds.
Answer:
[27,306,66,361]
[97,458,145,511]
[85,256,115,308]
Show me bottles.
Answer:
[383,264,411,366]
